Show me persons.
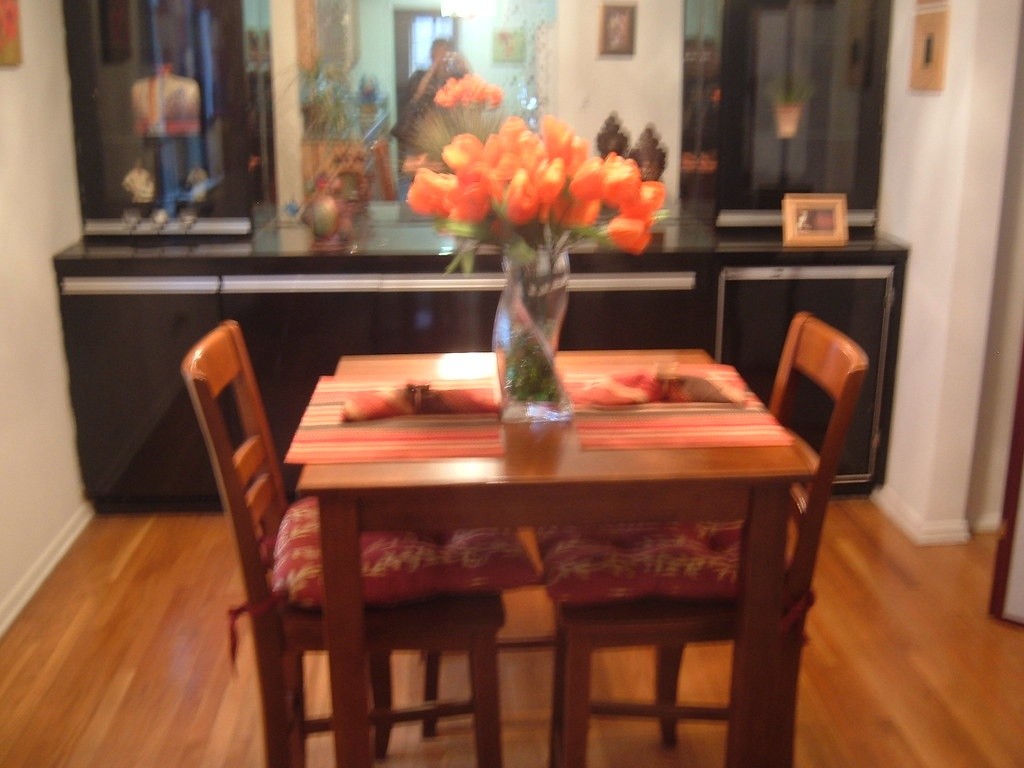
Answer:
[402,38,470,153]
[682,36,721,203]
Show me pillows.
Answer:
[535,525,742,603]
[270,495,543,608]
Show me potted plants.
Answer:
[759,76,816,140]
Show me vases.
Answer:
[492,249,573,424]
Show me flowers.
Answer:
[433,73,504,118]
[404,112,668,278]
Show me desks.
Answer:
[297,348,812,768]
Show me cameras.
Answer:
[442,52,457,75]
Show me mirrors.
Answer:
[269,0,683,226]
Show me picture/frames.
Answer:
[780,193,849,248]
[597,3,636,55]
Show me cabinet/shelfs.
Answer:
[62,0,278,245]
[50,197,911,512]
[678,0,893,243]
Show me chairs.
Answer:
[550,314,870,768]
[180,318,507,768]
